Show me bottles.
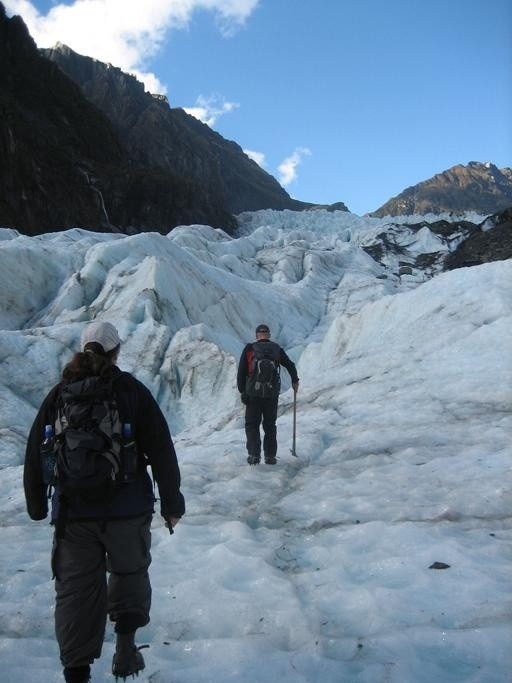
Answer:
[123,423,133,439]
[40,425,58,485]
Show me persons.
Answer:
[22,321,187,683]
[235,323,300,463]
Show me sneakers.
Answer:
[112,645,146,678]
[247,455,260,465]
[265,455,277,465]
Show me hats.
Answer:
[81,321,120,353]
[256,325,270,333]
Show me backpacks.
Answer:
[40,373,139,497]
[247,342,280,398]
